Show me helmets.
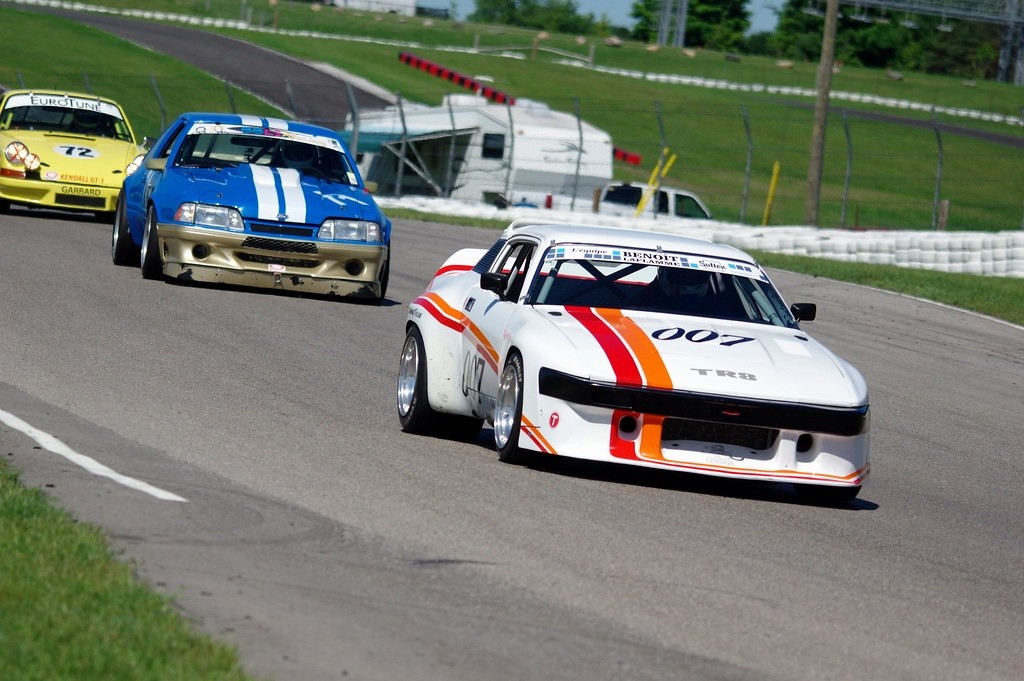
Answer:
[659,266,712,308]
[280,140,316,170]
[73,109,103,133]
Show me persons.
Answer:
[71,109,99,134]
[281,141,316,170]
[656,266,710,316]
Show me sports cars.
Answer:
[0,89,146,222]
[396,218,871,502]
[112,111,393,301]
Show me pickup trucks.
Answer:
[509,180,712,221]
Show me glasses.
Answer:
[675,280,710,298]
